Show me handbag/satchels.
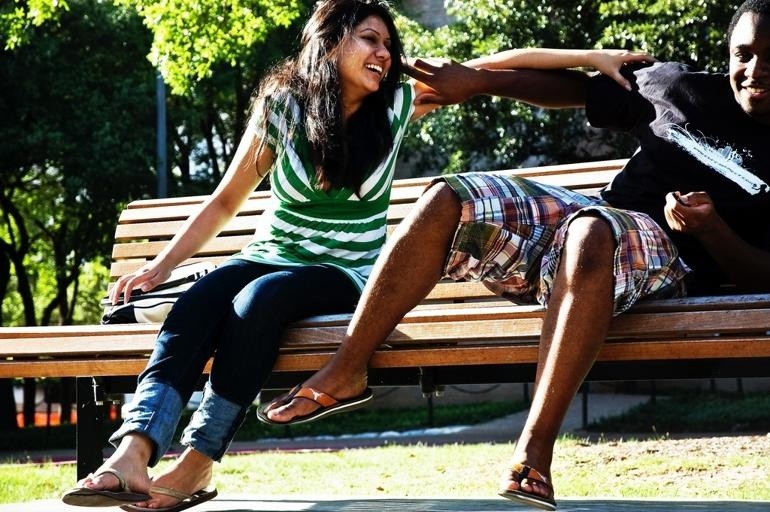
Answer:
[99,262,221,324]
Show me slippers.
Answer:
[61,468,218,512]
[497,463,557,511]
[256,382,374,426]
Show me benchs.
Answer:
[0,160,770,482]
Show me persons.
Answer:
[258,4,770,512]
[60,2,656,511]
